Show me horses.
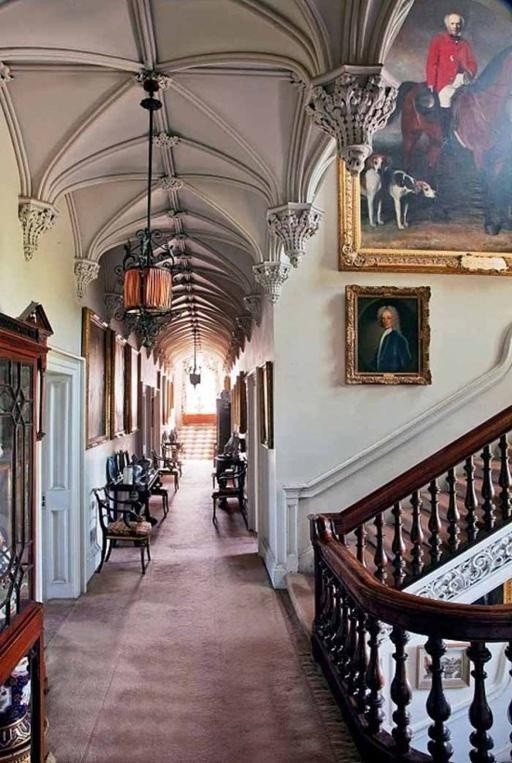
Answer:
[387,46,512,236]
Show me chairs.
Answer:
[94,430,249,577]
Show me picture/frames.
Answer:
[223,360,274,451]
[415,643,471,691]
[82,307,174,451]
[336,1,512,280]
[343,284,431,387]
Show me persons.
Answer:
[426,12,478,156]
[366,305,411,371]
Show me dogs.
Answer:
[383,169,438,230]
[360,153,391,228]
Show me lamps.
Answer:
[189,329,201,391]
[119,71,174,320]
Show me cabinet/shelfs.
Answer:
[1,298,52,763]
